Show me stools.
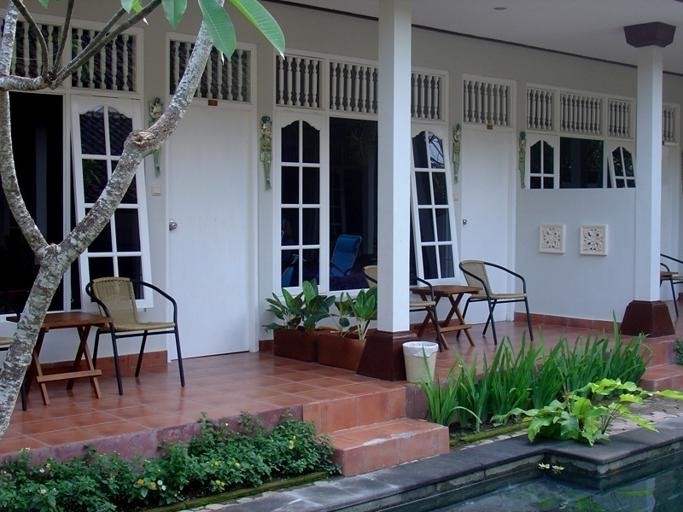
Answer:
[0,335,30,413]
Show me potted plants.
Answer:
[318,284,378,374]
[263,272,334,363]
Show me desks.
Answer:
[6,308,110,408]
[406,282,480,351]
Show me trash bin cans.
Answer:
[402,341,439,384]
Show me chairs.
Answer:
[456,259,535,346]
[364,264,444,355]
[314,230,366,283]
[81,274,188,394]
[659,251,683,322]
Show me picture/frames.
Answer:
[537,222,566,254]
[578,224,609,257]
